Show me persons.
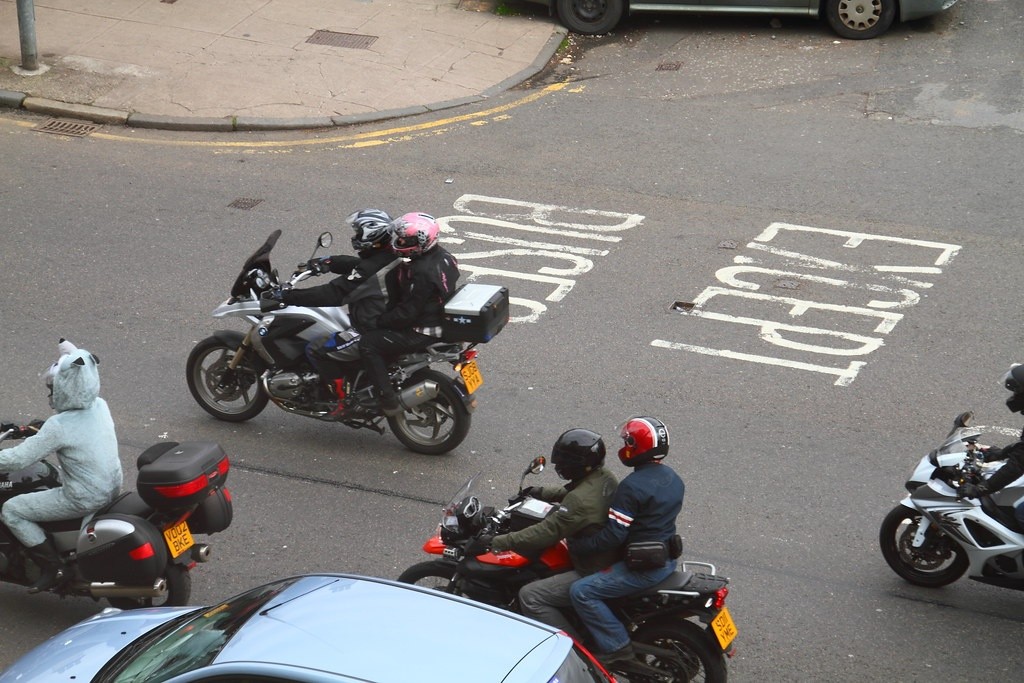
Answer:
[0,338,123,594]
[271,208,408,406]
[958,364,1024,500]
[470,429,619,639]
[568,413,684,663]
[360,212,460,412]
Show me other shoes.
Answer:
[591,644,636,664]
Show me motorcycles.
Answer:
[878,407,1024,596]
[0,418,234,608]
[184,228,511,455]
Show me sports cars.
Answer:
[395,454,736,683]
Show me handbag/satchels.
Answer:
[626,540,665,570]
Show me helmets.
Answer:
[45,362,59,410]
[551,428,606,480]
[618,415,670,467]
[1005,364,1024,413]
[391,212,440,257]
[352,208,394,251]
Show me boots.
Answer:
[358,365,399,410]
[27,534,66,595]
[320,377,361,422]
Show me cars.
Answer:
[0,571,622,683]
[531,0,964,41]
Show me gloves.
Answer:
[267,289,291,307]
[306,258,332,277]
[985,446,1003,463]
[956,483,983,500]
[517,486,534,494]
[25,418,45,437]
[475,533,493,551]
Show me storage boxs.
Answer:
[136,441,229,508]
[74,513,165,588]
[438,283,510,343]
[186,485,233,535]
